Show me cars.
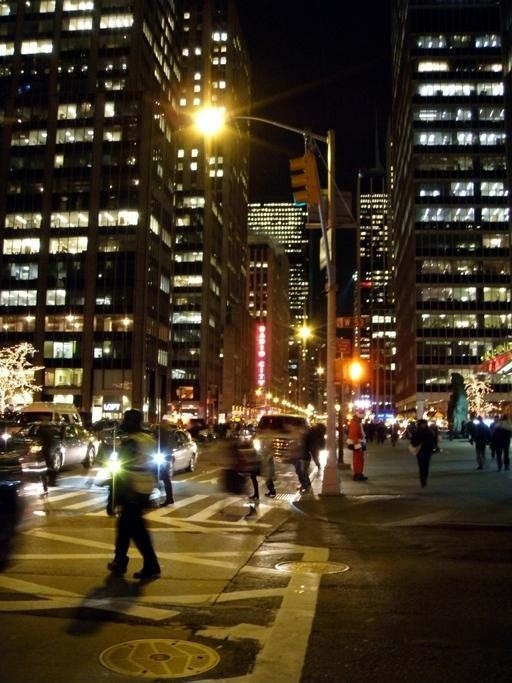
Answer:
[110,423,199,483]
[251,415,312,464]
[1,421,102,473]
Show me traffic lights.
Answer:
[287,152,320,203]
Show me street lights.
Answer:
[193,109,340,496]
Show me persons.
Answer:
[35,416,57,495]
[107,408,160,578]
[154,424,174,506]
[228,408,512,499]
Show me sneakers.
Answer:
[159,498,174,505]
[353,474,367,481]
[249,489,276,498]
[133,563,160,579]
[108,560,128,574]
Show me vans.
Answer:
[1,403,85,438]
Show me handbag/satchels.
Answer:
[408,444,423,456]
